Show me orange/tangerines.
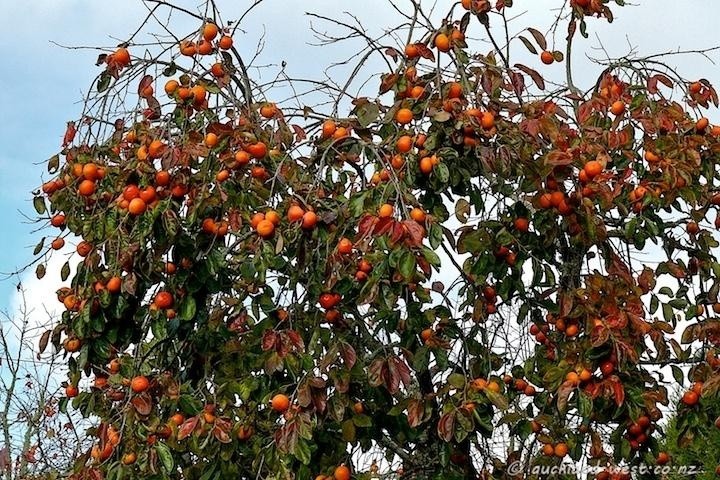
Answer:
[42,0,720,480]
